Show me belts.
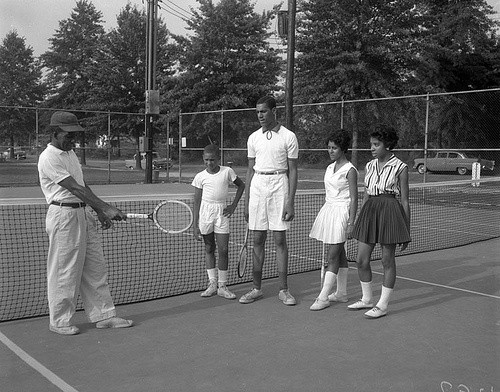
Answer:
[255,170,287,175]
[51,200,86,208]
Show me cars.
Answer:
[413,150,496,175]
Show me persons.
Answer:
[192,144,245,300]
[348,125,410,318]
[245,95,299,305]
[308,129,360,311]
[37,111,134,336]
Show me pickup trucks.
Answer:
[125,152,173,170]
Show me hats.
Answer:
[49,110,85,132]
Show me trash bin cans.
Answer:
[227,162,233,168]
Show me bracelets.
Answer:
[347,222,353,226]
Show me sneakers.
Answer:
[48,324,80,335]
[328,292,348,303]
[363,306,388,318]
[200,286,217,297]
[217,287,236,299]
[279,288,296,305]
[348,299,373,309]
[309,298,330,311]
[95,317,133,329]
[238,288,264,303]
